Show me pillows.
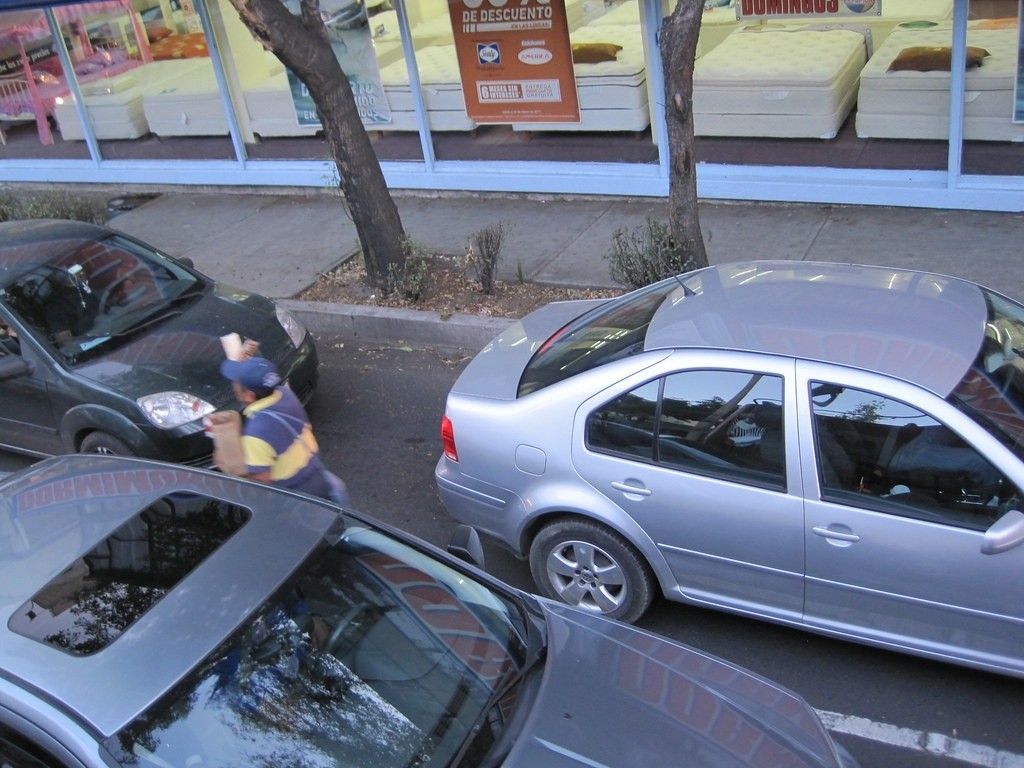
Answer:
[886,46,991,71]
[572,43,623,64]
[146,28,171,42]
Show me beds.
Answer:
[855,19,1024,143]
[0,0,327,146]
[511,0,738,140]
[692,24,873,140]
[364,0,483,137]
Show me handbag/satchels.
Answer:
[321,469,350,509]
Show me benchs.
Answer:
[606,374,756,457]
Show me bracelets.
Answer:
[309,611,324,621]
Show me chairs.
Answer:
[819,406,946,504]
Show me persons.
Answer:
[44,260,133,349]
[219,338,331,499]
[851,396,1000,485]
[251,595,330,666]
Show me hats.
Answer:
[219,357,284,398]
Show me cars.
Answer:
[434,261,1023,678]
[0,453,861,768]
[1,219,319,456]
